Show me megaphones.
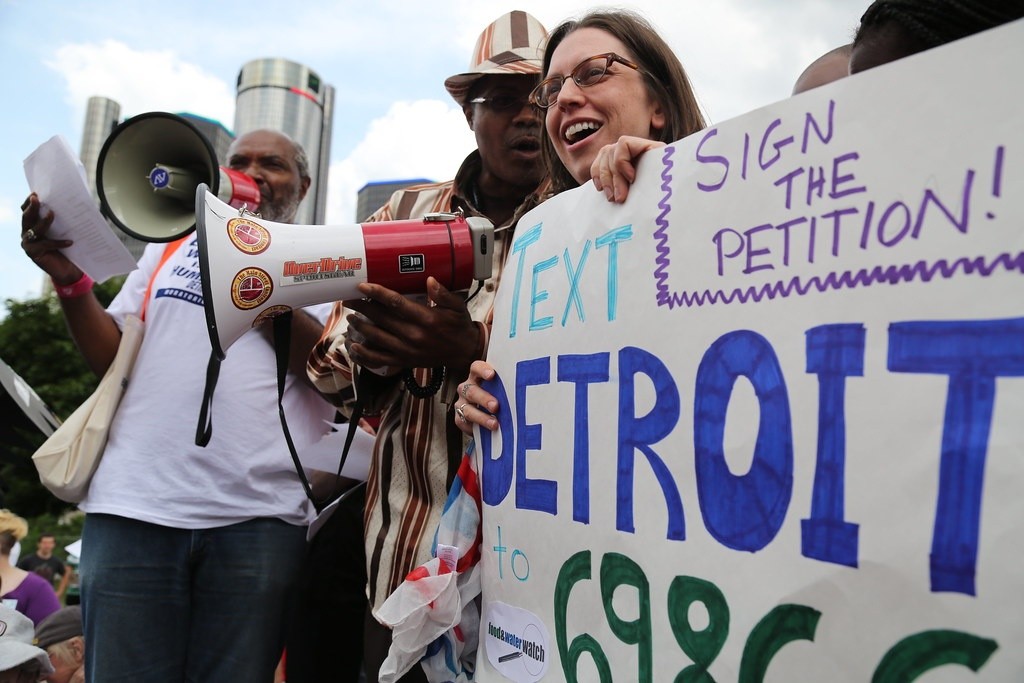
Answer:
[95,112,261,243]
[195,182,494,362]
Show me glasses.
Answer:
[470,92,534,115]
[529,52,668,109]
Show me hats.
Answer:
[35,606,84,648]
[445,11,552,106]
[0,604,56,675]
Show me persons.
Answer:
[303,11,557,683]
[792,0,1024,95]
[454,13,708,437]
[0,508,88,683]
[20,127,336,683]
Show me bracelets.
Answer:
[51,274,95,299]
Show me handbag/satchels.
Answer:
[31,234,190,505]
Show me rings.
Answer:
[456,404,470,424]
[27,229,37,240]
[462,384,476,398]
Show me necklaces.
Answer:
[471,172,480,212]
